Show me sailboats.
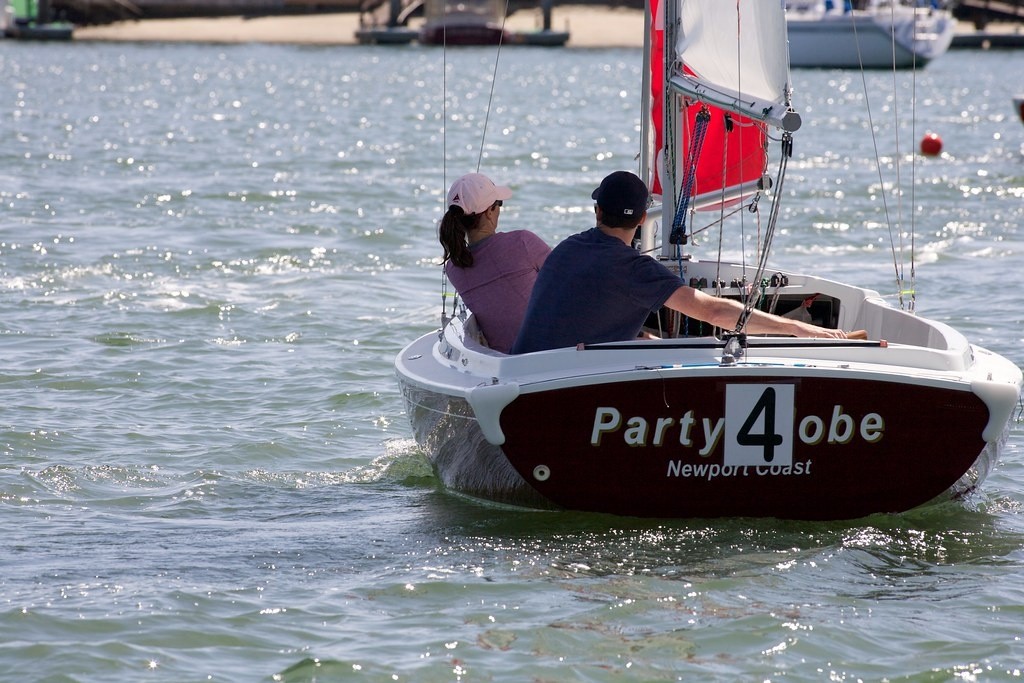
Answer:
[391,0,1024,523]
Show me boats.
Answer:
[353,0,573,48]
[0,0,74,43]
[782,0,958,70]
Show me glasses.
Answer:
[490,200,503,208]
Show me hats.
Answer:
[592,171,648,217]
[447,174,513,215]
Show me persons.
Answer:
[439,173,551,355]
[509,170,847,355]
